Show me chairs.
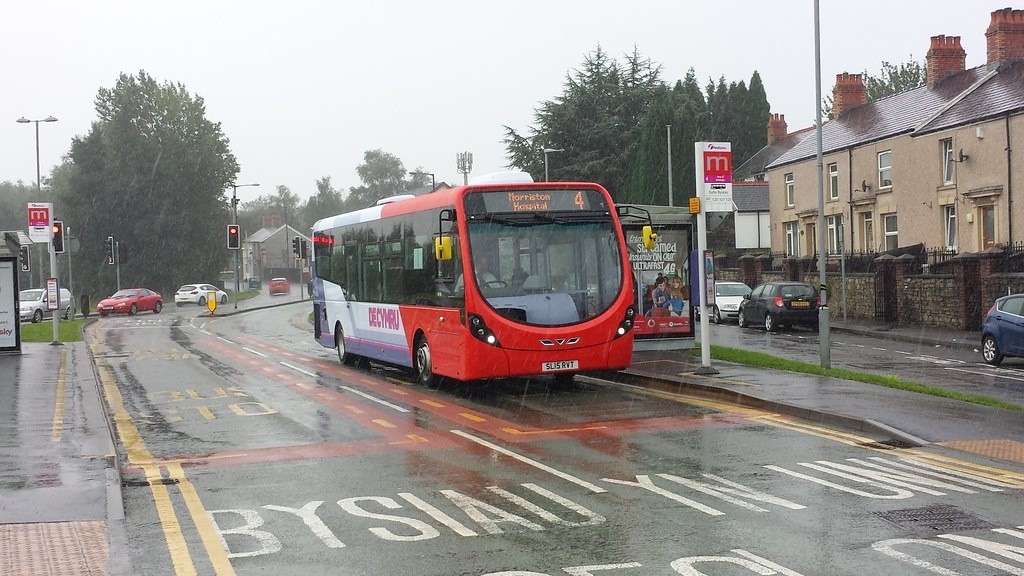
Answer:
[791,288,800,296]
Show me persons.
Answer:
[454,254,501,297]
[644,273,689,317]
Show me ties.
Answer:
[478,274,491,290]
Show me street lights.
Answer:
[408,172,435,192]
[226,183,260,309]
[15,115,59,288]
[544,147,565,183]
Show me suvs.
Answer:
[19,288,76,324]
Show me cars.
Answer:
[738,281,820,333]
[981,294,1024,366]
[96,288,163,317]
[269,277,290,297]
[695,281,754,323]
[175,284,228,307]
[249,278,262,289]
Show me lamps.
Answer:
[958,149,969,163]
[800,230,804,235]
[862,180,871,192]
[976,127,985,139]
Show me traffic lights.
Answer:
[227,225,240,249]
[19,245,31,271]
[105,236,114,264]
[48,221,65,254]
[292,236,302,261]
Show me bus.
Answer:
[310,181,657,390]
